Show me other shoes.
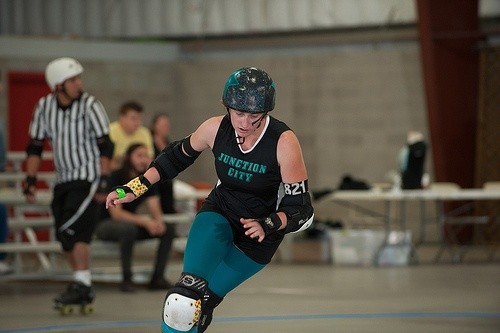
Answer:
[146,277,176,289]
[119,281,136,292]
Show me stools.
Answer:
[435,215,496,262]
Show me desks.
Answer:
[318,188,500,265]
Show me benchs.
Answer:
[1,151,196,279]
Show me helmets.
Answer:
[223,66,276,112]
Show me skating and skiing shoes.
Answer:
[53,279,95,315]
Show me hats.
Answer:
[44,56,84,89]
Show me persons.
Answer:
[106,67,315,333]
[22,57,115,315]
[109,101,155,172]
[150,112,177,214]
[96,142,176,292]
